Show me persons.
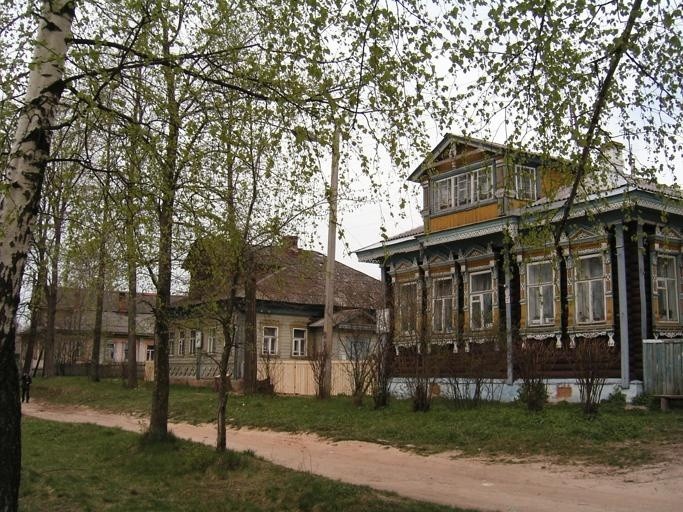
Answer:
[21,371,32,403]
